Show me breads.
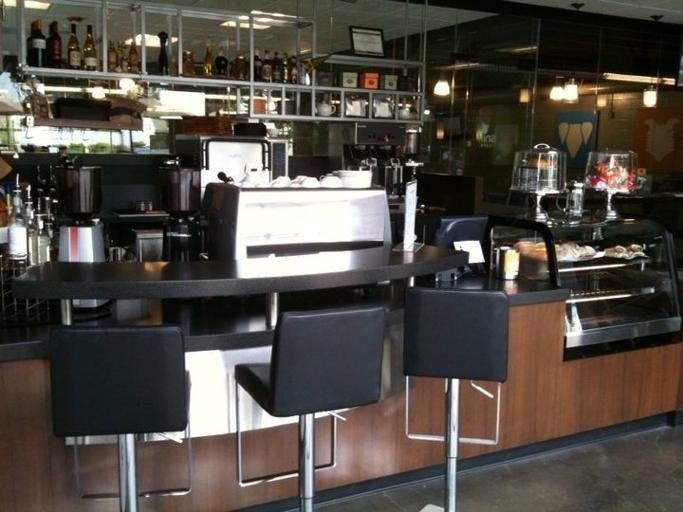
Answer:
[508,239,648,262]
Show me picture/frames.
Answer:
[348,25,385,58]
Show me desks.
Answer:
[6,241,469,326]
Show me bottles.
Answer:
[45,216,56,260]
[122,44,128,73]
[115,39,122,71]
[34,203,49,264]
[26,17,48,68]
[280,50,290,83]
[201,40,216,78]
[261,48,272,84]
[157,31,169,75]
[5,185,29,262]
[82,22,99,71]
[253,45,262,82]
[215,45,228,76]
[108,37,117,71]
[46,18,63,69]
[298,59,307,86]
[271,48,282,83]
[65,21,82,69]
[127,38,140,73]
[289,53,298,83]
[22,194,38,268]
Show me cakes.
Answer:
[584,157,637,195]
[511,149,567,195]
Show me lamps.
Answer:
[563,79,579,105]
[548,78,563,101]
[433,71,451,97]
[641,84,657,108]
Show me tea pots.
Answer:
[313,99,336,117]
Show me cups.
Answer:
[497,245,517,280]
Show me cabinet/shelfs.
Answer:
[14,2,427,129]
[482,217,682,361]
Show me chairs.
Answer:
[47,325,192,512]
[403,288,508,512]
[232,306,385,512]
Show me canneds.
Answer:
[228,51,250,81]
[179,47,197,78]
